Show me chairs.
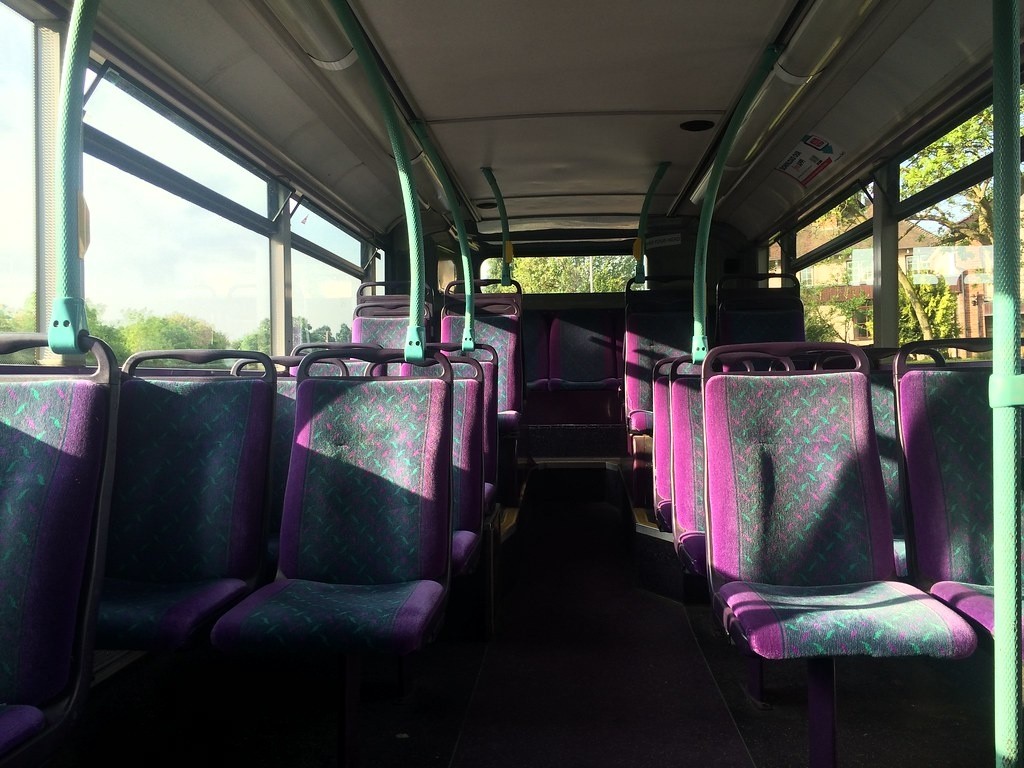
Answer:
[0,272,1024,768]
